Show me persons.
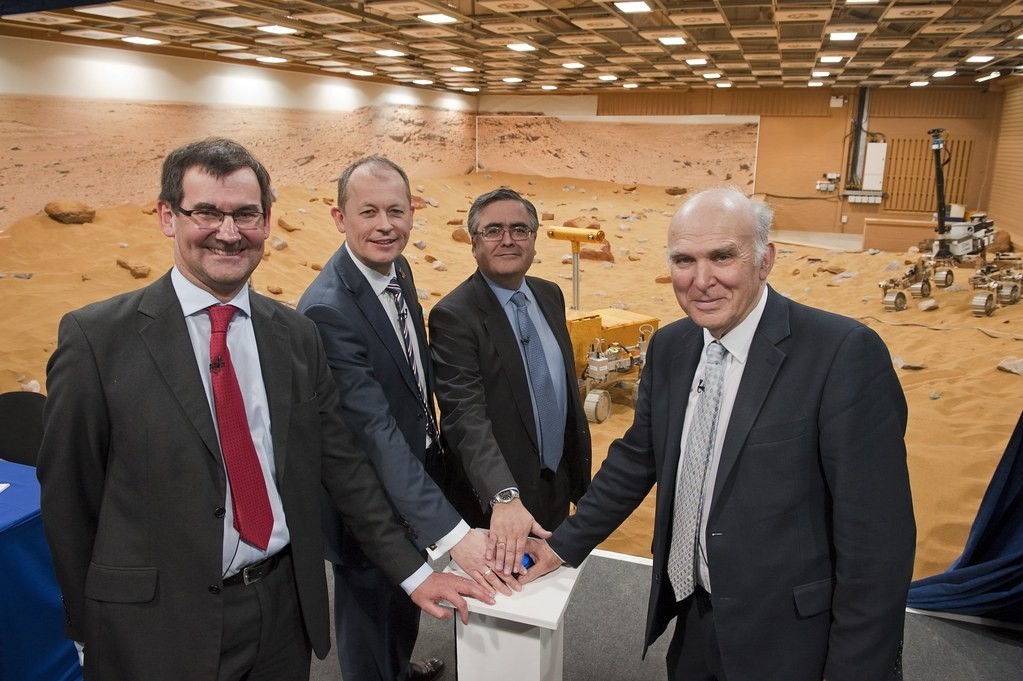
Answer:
[36,138,496,681]
[520,188,917,681]
[428,188,591,577]
[296,157,525,681]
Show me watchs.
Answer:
[491,490,519,506]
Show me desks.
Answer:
[0,458,84,681]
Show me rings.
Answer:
[498,541,505,544]
[484,570,491,575]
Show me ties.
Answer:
[508,291,564,475]
[666,342,729,602]
[385,278,446,455]
[201,304,275,552]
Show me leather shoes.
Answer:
[409,655,445,681]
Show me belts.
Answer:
[220,543,294,587]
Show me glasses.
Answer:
[473,224,536,241]
[170,200,267,230]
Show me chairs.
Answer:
[0,391,48,467]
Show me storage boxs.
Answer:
[943,203,966,218]
[932,231,995,257]
